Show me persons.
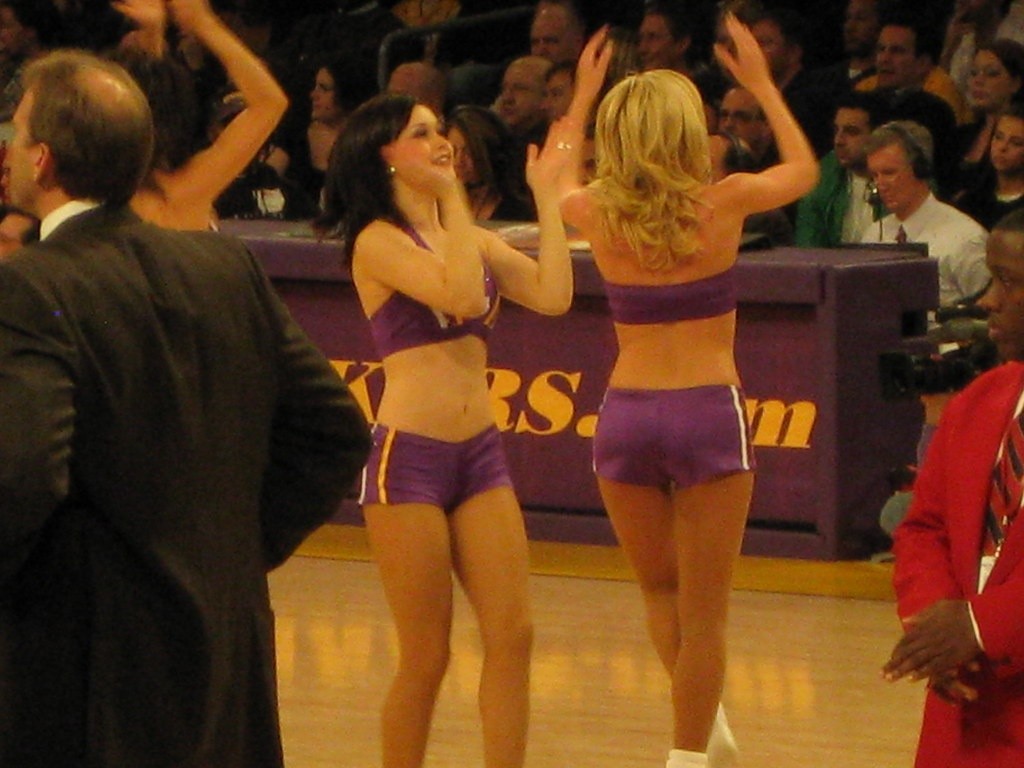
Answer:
[560,15,821,768]
[0,0,1023,360]
[0,51,374,767]
[883,207,1023,767]
[313,94,574,768]
[113,0,287,232]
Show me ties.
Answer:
[893,223,907,244]
[981,406,1024,557]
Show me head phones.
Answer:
[720,130,756,175]
[889,122,933,180]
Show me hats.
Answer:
[216,92,247,121]
[882,119,934,166]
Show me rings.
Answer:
[557,143,572,149]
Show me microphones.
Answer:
[868,193,881,206]
[939,316,991,340]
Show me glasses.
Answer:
[719,108,767,123]
